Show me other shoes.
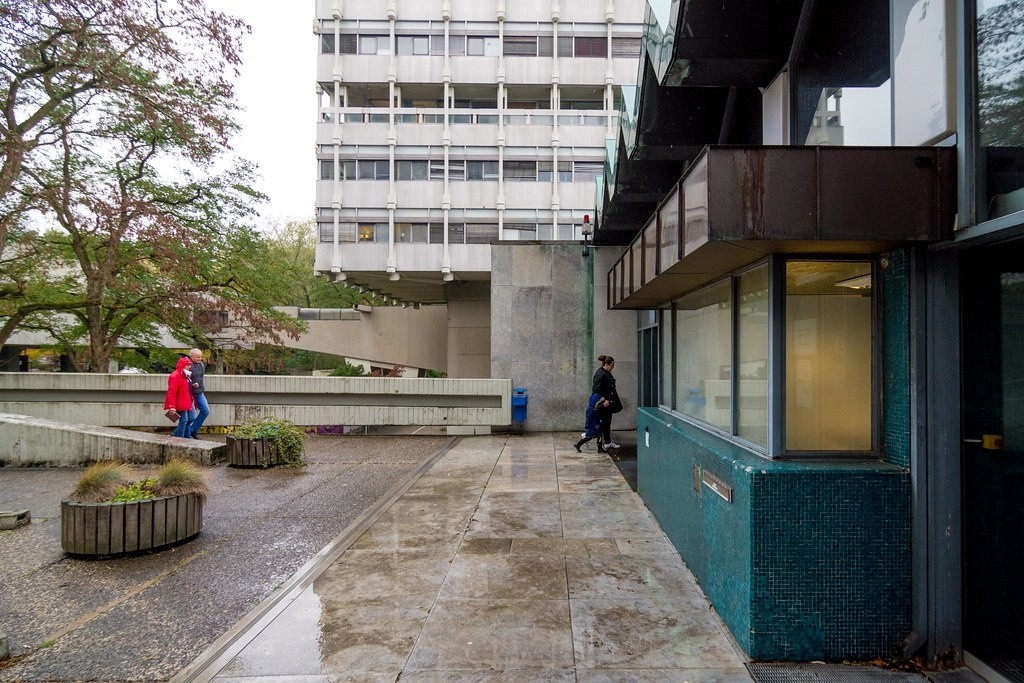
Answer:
[581,433,588,443]
[598,447,609,453]
[604,442,621,449]
[574,444,583,452]
[191,431,200,440]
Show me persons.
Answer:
[573,393,609,454]
[184,348,209,440]
[163,357,199,439]
[581,355,623,449]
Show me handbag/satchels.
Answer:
[612,390,623,413]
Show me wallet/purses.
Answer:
[165,410,181,423]
